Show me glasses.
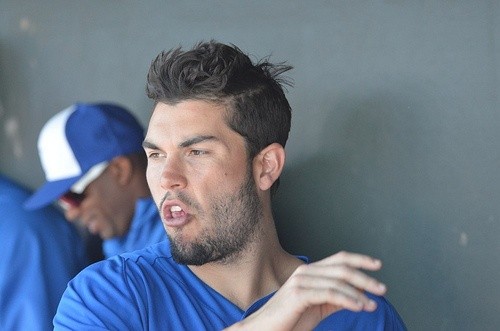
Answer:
[60,159,110,210]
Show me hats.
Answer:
[23,101,145,211]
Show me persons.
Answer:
[0,175,94,331]
[22,102,172,261]
[52,40,407,331]
